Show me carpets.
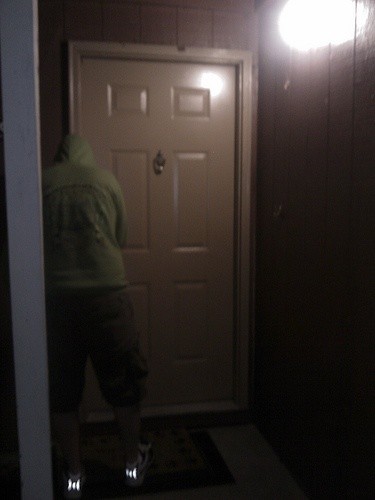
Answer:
[51,414,238,499]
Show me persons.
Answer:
[44,132,152,499]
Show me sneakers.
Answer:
[126,440,155,486]
[65,462,86,498]
